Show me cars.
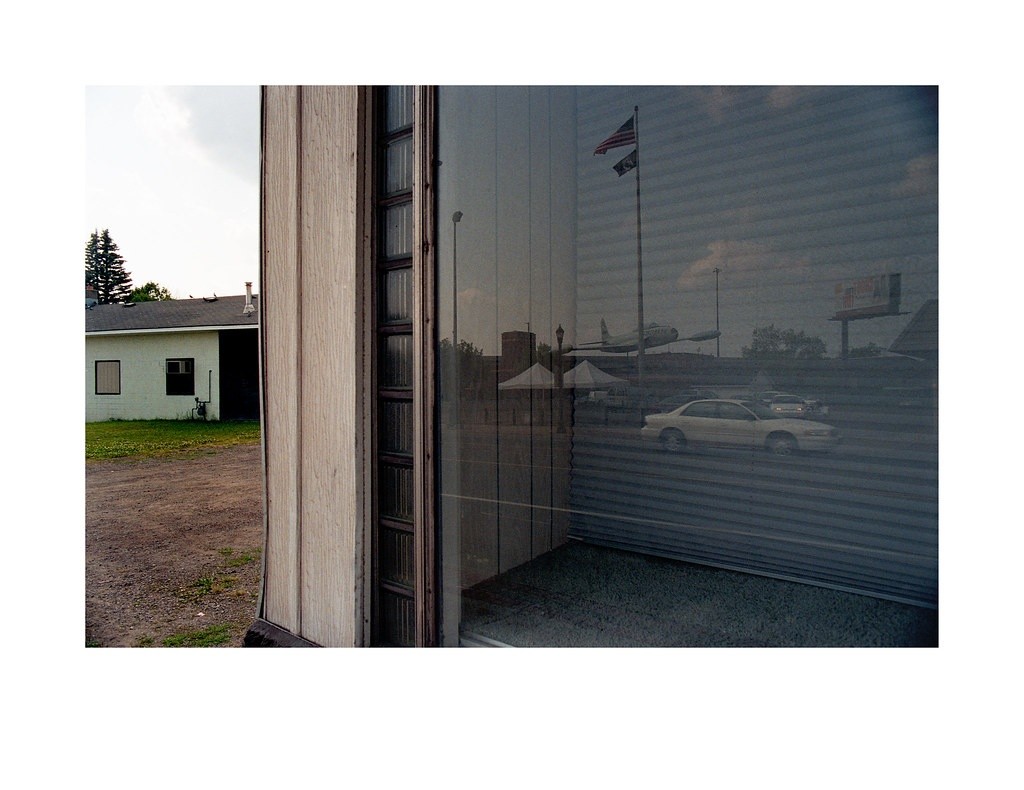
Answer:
[592,384,721,413]
[801,398,831,418]
[759,388,783,407]
[639,398,845,459]
[770,393,808,419]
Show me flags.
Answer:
[592,116,636,154]
[613,149,638,177]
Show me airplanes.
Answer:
[565,318,722,351]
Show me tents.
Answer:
[498,359,631,427]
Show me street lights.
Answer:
[554,324,568,433]
[446,209,464,435]
